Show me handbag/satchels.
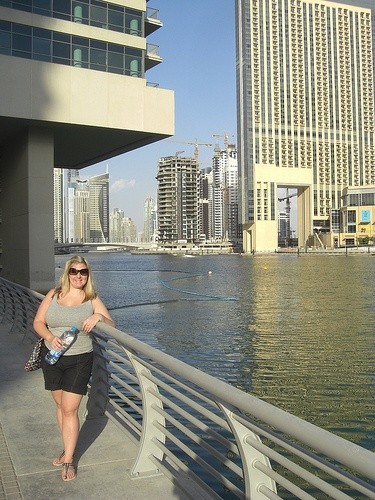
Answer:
[23,336,44,372]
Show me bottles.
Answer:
[45,326,78,365]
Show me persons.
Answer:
[32,254,116,482]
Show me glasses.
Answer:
[69,268,90,277]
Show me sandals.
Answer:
[52,449,76,466]
[61,463,77,481]
